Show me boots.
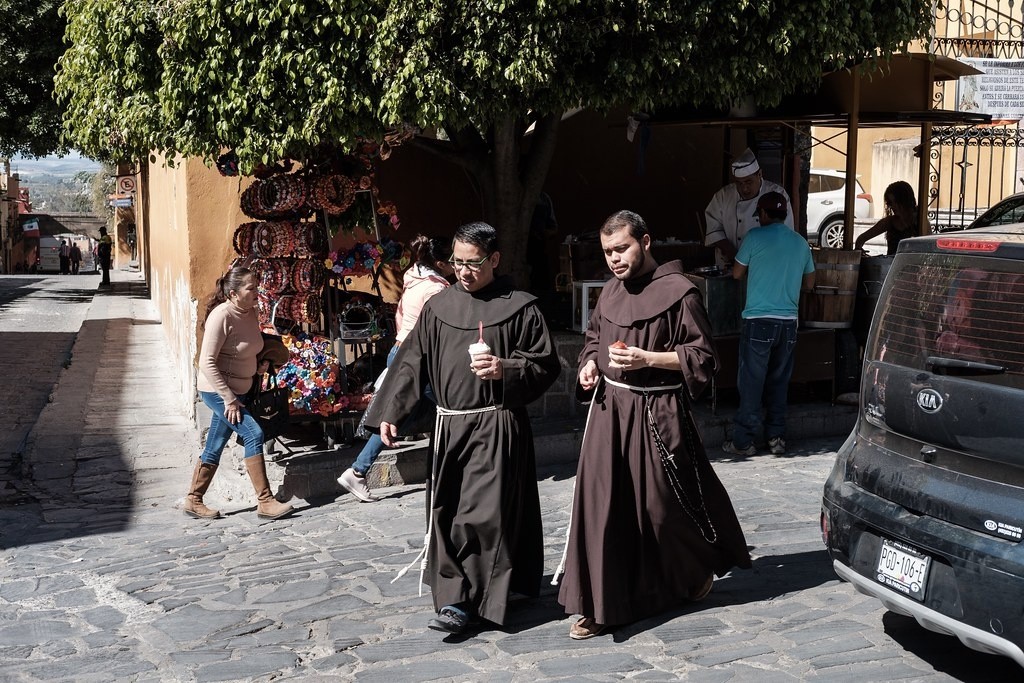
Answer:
[244,453,295,520]
[183,455,220,519]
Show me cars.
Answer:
[819,226,1024,671]
[806,168,873,248]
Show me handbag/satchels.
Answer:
[235,358,292,448]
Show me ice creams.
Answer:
[609,340,628,349]
[469,342,491,352]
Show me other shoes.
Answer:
[427,605,484,636]
[100,281,110,286]
[688,569,715,602]
[73,271,77,274]
[569,617,610,640]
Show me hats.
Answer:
[752,190,787,218]
[731,147,760,178]
[99,226,106,231]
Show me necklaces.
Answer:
[227,173,377,334]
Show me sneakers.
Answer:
[336,467,379,503]
[767,436,787,455]
[722,440,757,457]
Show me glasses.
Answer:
[447,252,492,271]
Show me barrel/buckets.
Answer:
[804,250,862,328]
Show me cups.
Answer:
[607,345,629,365]
[468,343,491,370]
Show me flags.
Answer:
[22,217,40,237]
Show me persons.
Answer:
[558,210,750,640]
[854,180,931,256]
[185,266,294,521]
[58,240,71,274]
[92,227,112,286]
[68,243,82,275]
[363,222,562,634]
[704,148,794,265]
[337,232,455,502]
[721,191,817,457]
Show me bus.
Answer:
[36,236,72,272]
